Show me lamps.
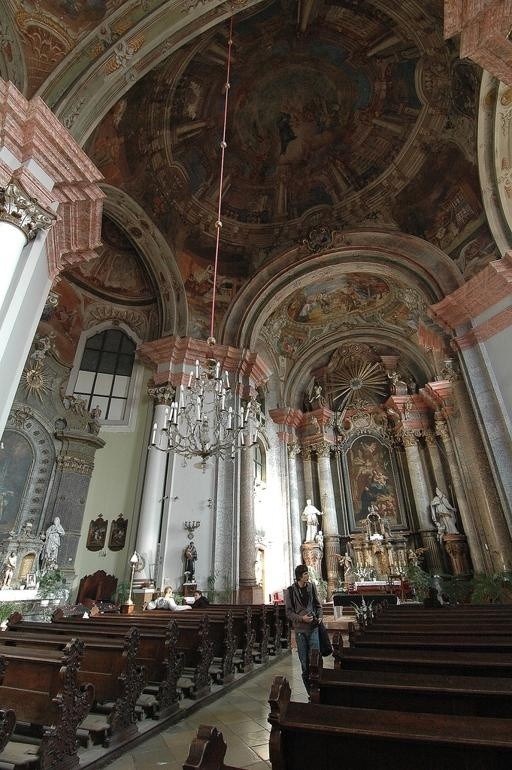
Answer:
[150,11,259,465]
[125,551,139,604]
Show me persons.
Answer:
[435,522,447,545]
[283,562,326,699]
[185,545,193,560]
[408,548,423,571]
[145,586,193,612]
[386,370,408,388]
[191,590,210,609]
[300,498,327,545]
[338,552,354,577]
[315,531,326,552]
[422,587,442,611]
[431,487,461,536]
[2,551,18,587]
[307,385,324,403]
[184,541,198,581]
[40,516,65,572]
[349,439,399,522]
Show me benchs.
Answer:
[183,722,245,770]
[268,676,511,769]
[301,602,512,717]
[0,601,291,770]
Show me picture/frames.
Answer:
[25,574,36,588]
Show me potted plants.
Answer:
[35,567,72,601]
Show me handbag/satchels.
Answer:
[318,620,333,657]
